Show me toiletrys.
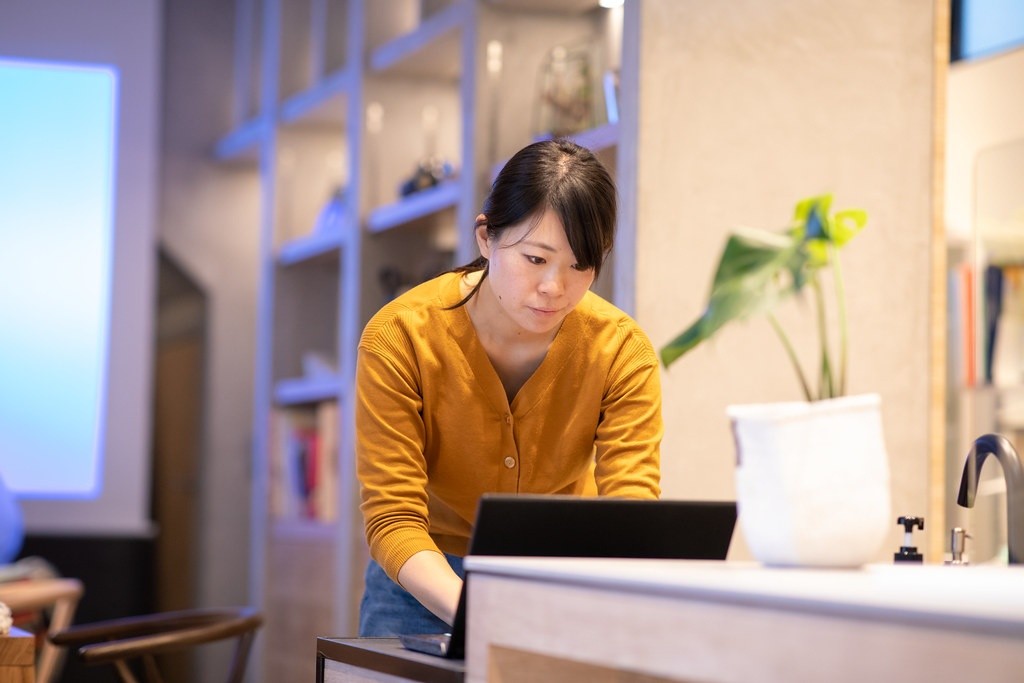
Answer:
[894,516,926,562]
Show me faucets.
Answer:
[956,433,1024,565]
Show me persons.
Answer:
[344,138,669,638]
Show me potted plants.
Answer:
[659,194,895,566]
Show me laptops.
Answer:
[398,493,738,661]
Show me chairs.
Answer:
[48,603,262,683]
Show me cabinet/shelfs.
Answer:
[212,0,1024,683]
